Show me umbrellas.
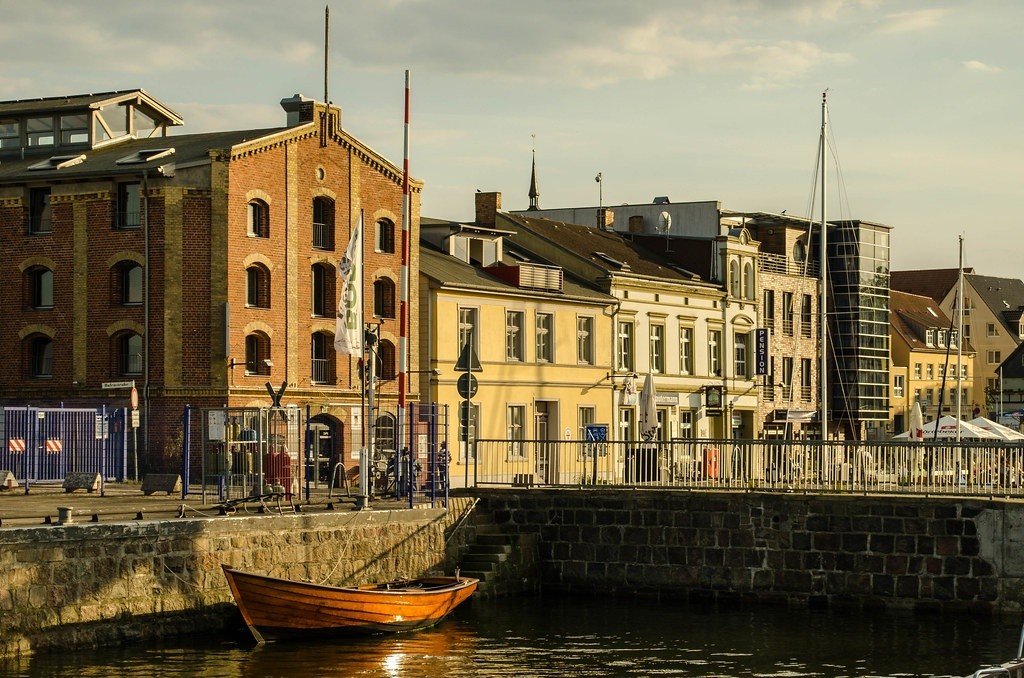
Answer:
[638,364,659,444]
[904,403,926,461]
[890,414,1024,466]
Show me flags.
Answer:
[333,213,364,359]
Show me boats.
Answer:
[221,563,480,645]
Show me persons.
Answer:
[438,440,452,490]
